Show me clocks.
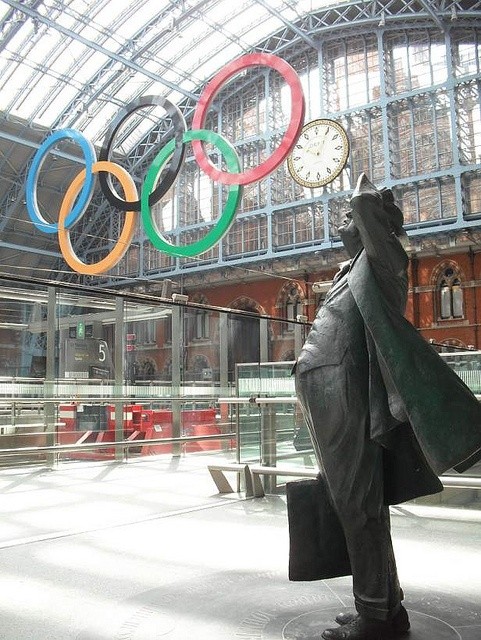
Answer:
[286,117,350,189]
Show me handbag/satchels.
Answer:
[285,470,352,582]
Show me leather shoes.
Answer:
[334,605,409,633]
[320,613,402,640]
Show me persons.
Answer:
[287,174,481,639]
